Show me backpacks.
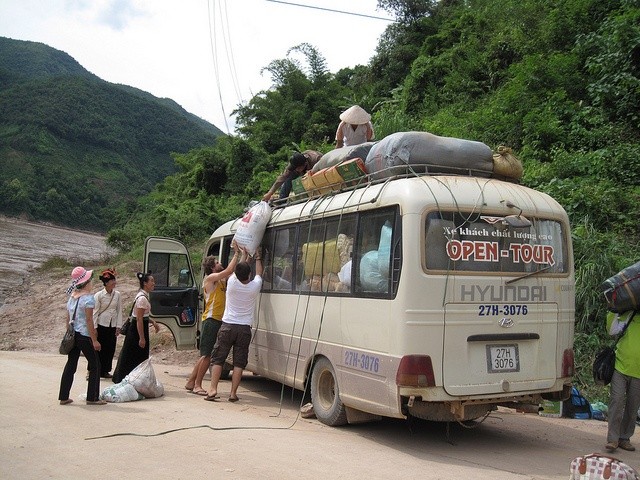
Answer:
[560,384,593,420]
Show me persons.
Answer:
[605,308,640,450]
[112,272,160,384]
[262,150,323,210]
[185,239,239,396]
[92,267,121,377]
[58,268,108,405]
[335,106,375,150]
[204,246,263,401]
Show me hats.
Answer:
[339,104,372,125]
[285,152,306,172]
[98,265,117,283]
[65,266,93,294]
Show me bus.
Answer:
[142,164,575,426]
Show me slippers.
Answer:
[204,394,221,401]
[229,395,239,402]
[192,389,209,396]
[185,386,194,390]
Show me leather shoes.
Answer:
[60,398,73,405]
[101,372,114,378]
[605,441,619,449]
[619,438,635,451]
[86,374,89,380]
[86,400,107,405]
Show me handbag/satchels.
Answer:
[569,452,640,479]
[591,345,616,387]
[119,317,131,335]
[58,322,76,355]
